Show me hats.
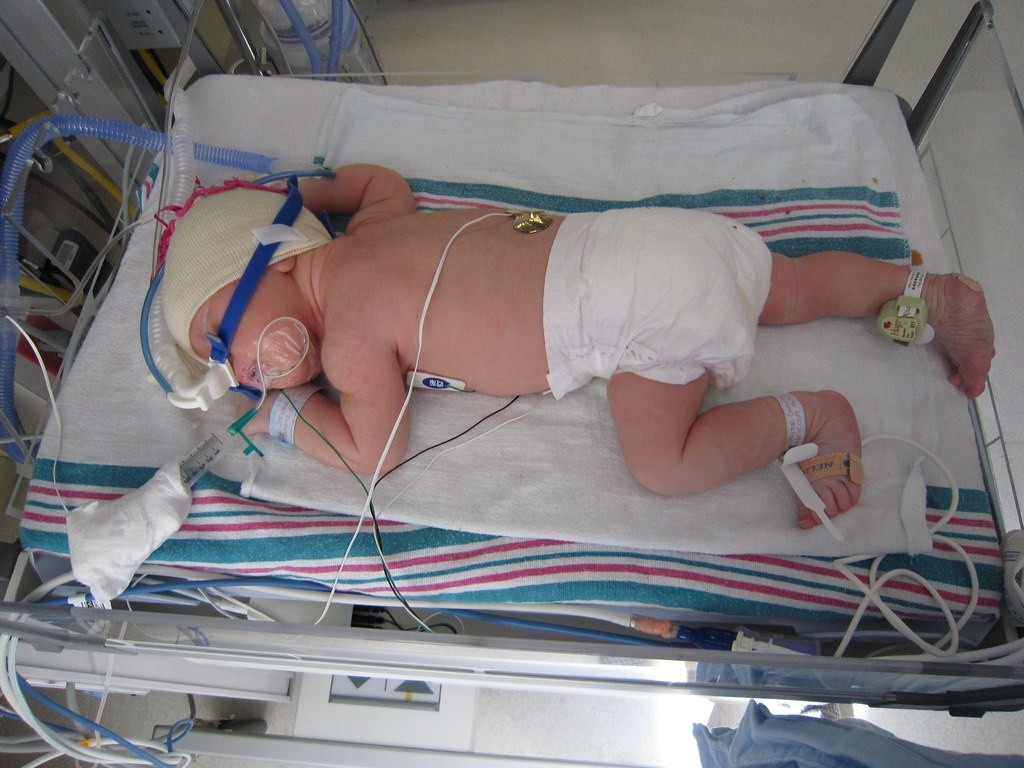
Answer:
[162,189,334,364]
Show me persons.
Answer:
[161,164,995,528]
